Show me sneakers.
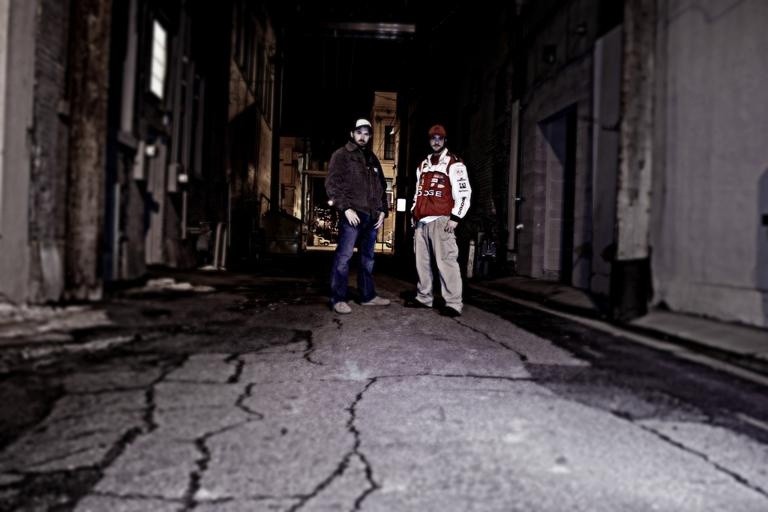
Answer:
[404,298,432,308]
[330,302,351,314]
[361,296,390,306]
[439,306,460,316]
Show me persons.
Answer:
[405,123,473,317]
[318,215,332,236]
[322,118,390,314]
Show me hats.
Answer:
[352,118,371,132]
[429,124,445,138]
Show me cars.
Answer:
[317,237,329,246]
